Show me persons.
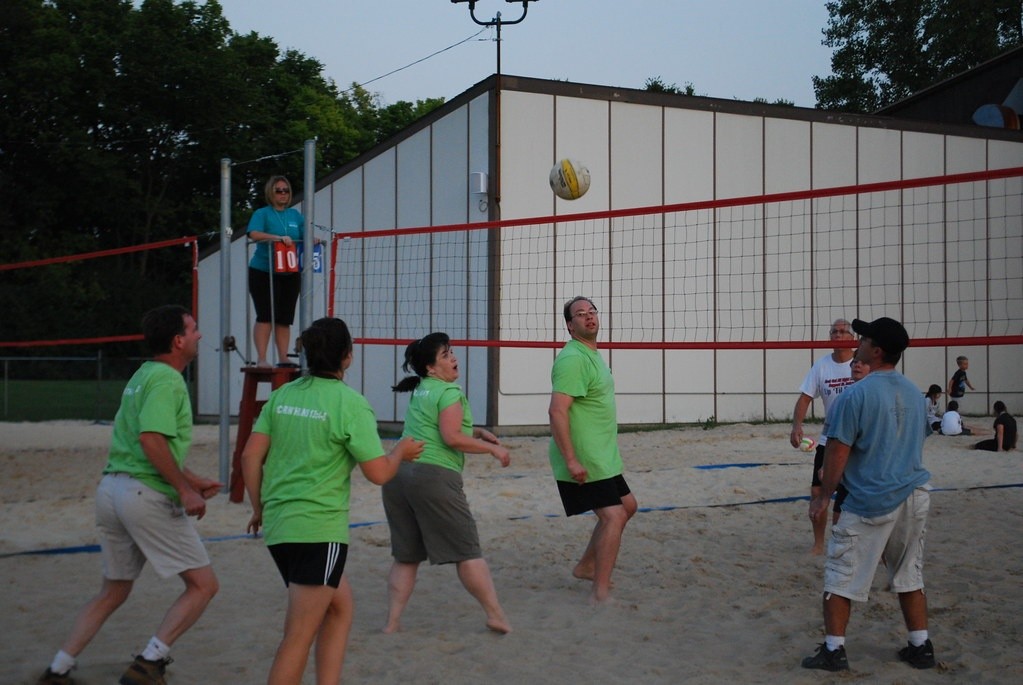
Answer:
[549,297,638,605]
[924,384,942,431]
[792,319,871,559]
[801,317,934,672]
[974,401,1017,452]
[938,400,994,436]
[40,308,225,685]
[247,175,320,368]
[240,317,426,685]
[382,332,515,634]
[948,356,976,412]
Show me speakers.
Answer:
[471,172,488,194]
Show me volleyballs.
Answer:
[800,436,815,452]
[549,158,592,200]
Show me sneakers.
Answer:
[36,666,75,685]
[899,638,935,669]
[801,640,849,672]
[119,654,174,685]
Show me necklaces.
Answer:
[273,208,290,236]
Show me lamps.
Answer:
[469,171,488,194]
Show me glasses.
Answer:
[272,188,289,193]
[828,328,854,335]
[571,310,598,318]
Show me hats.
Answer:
[851,317,909,353]
